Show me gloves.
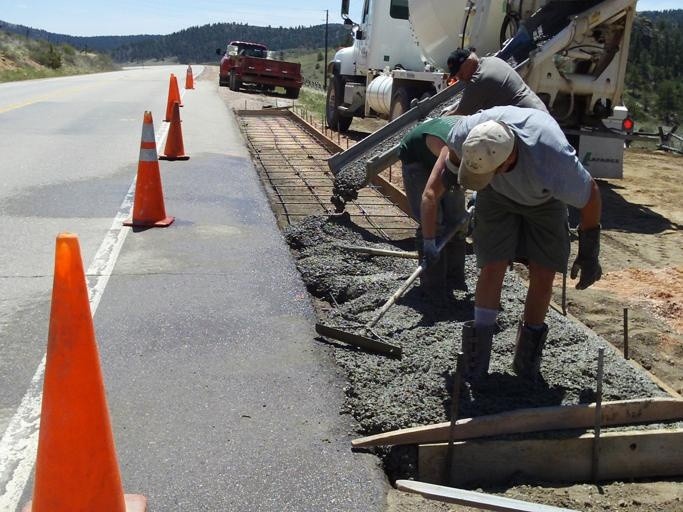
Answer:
[571,225,603,292]
[421,237,440,268]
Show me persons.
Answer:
[419,105,605,400]
[443,45,551,117]
[399,113,480,324]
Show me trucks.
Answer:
[215,40,304,100]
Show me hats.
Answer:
[457,119,513,190]
[448,48,472,78]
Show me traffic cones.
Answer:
[184,64,195,89]
[159,101,190,161]
[21,232,148,511]
[123,110,176,229]
[162,72,184,122]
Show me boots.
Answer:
[511,319,550,390]
[460,320,503,393]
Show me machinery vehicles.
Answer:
[325,1,638,179]
